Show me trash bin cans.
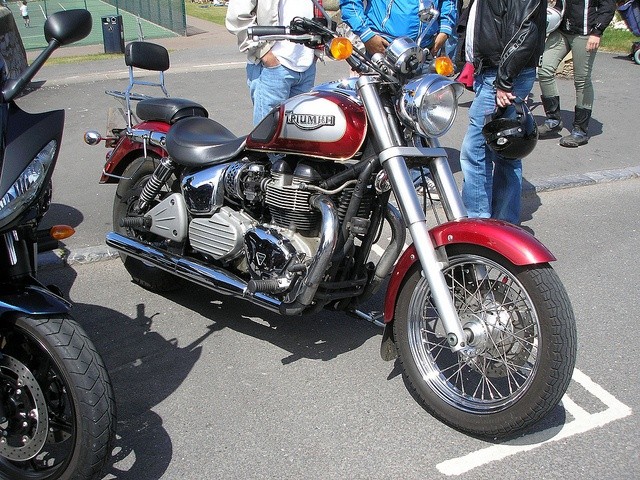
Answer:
[101,15,125,54]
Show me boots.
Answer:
[537,95,563,136]
[559,106,592,147]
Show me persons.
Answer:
[340,0,458,201]
[225,1,324,130]
[538,0,617,147]
[455,0,547,225]
[20,0,30,28]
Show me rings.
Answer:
[502,96,507,100]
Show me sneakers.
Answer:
[416,177,441,200]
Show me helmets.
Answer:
[546,1,565,33]
[481,100,539,160]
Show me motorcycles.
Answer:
[0,9,117,480]
[83,0,578,438]
